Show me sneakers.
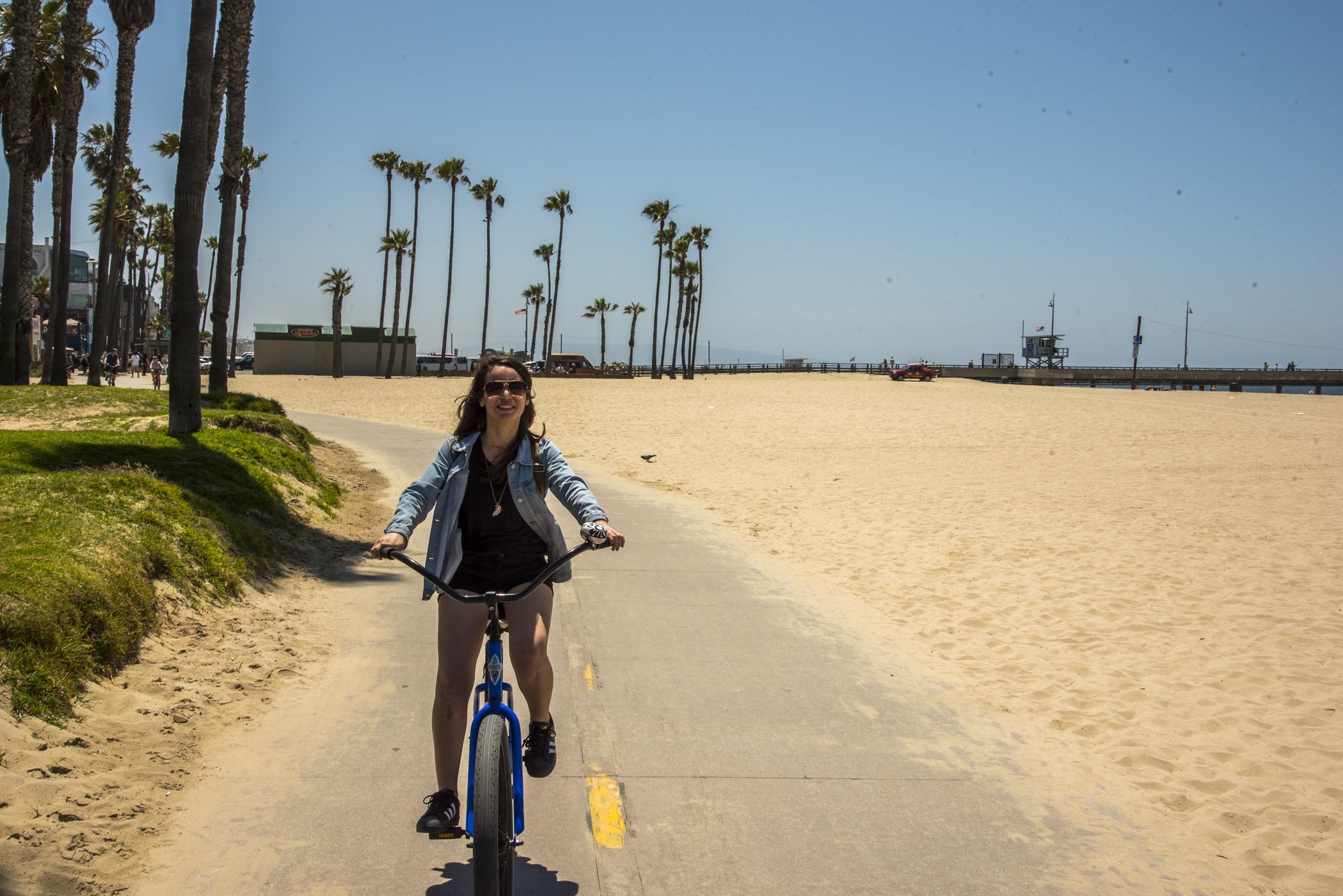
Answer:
[520,714,558,776]
[414,789,458,831]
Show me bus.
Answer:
[416,355,468,372]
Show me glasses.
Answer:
[481,379,527,398]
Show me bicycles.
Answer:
[151,367,162,390]
[380,537,611,896]
[106,362,117,386]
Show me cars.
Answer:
[199,352,254,375]
[522,360,545,372]
[76,355,90,370]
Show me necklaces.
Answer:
[481,435,508,517]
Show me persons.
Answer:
[1264,362,1269,371]
[130,351,140,378]
[71,353,76,373]
[556,364,564,375]
[66,352,74,379]
[1291,362,1296,371]
[104,348,120,386]
[1149,386,1167,391]
[80,351,89,373]
[128,352,161,371]
[969,360,974,368]
[141,353,149,376]
[890,357,894,368]
[1275,363,1279,370]
[150,356,164,380]
[1287,363,1291,371]
[372,356,623,839]
[570,363,576,373]
[881,359,888,373]
[160,355,168,375]
[470,360,475,372]
[100,351,107,363]
[705,365,708,369]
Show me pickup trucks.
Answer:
[889,365,937,382]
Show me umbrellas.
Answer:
[52,346,74,351]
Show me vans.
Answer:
[544,353,603,375]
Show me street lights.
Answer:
[1183,299,1193,369]
[86,257,98,321]
[1048,292,1055,366]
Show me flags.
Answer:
[514,309,526,314]
[850,357,855,362]
[1036,326,1044,331]
